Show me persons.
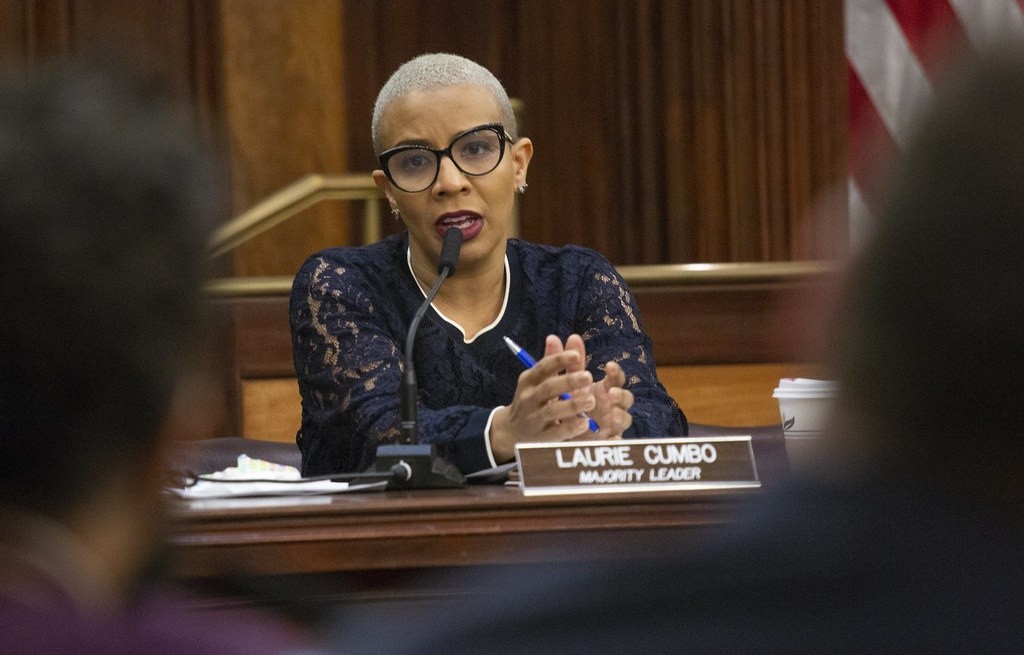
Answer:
[405,41,1024,654]
[287,50,689,482]
[0,49,331,655]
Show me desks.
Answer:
[141,472,766,613]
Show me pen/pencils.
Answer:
[503,334,601,434]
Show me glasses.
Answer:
[377,123,515,194]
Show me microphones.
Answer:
[346,226,468,490]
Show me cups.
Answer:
[772,378,843,478]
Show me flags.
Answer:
[846,0,1024,360]
